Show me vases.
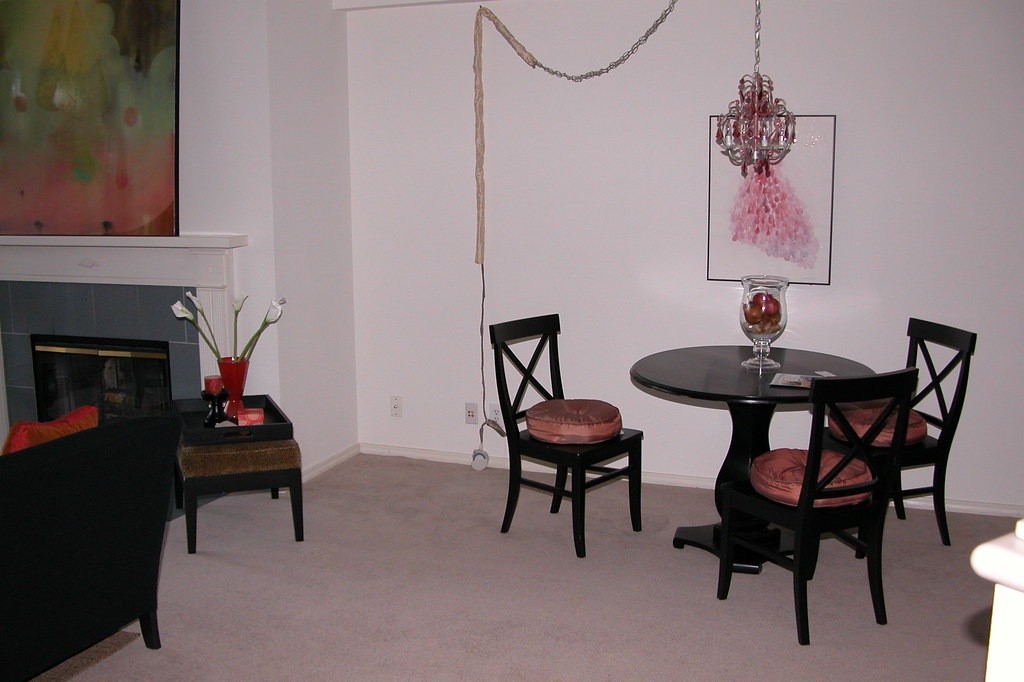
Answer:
[217,357,251,419]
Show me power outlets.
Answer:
[489,404,505,429]
[465,404,478,424]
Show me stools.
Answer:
[169,394,305,555]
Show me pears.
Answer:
[743,290,780,323]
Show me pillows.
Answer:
[1,406,98,454]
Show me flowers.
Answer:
[172,291,286,361]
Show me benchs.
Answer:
[0,412,182,682]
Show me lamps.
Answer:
[715,0,796,177]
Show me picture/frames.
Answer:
[707,114,836,287]
[0,0,180,238]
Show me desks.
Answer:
[629,346,878,575]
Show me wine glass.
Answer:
[738,274,791,374]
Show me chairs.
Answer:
[717,368,919,645]
[489,314,645,556]
[818,316,977,558]
